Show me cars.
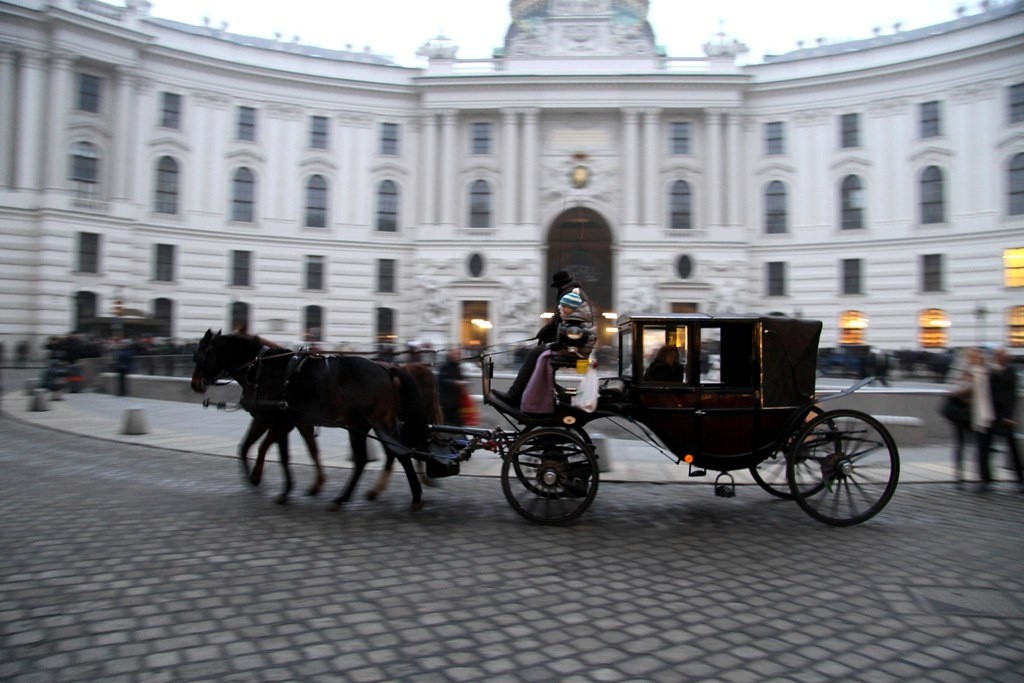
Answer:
[821,342,1024,387]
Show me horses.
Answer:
[189,323,443,514]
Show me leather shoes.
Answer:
[493,388,515,408]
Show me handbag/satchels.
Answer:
[939,398,970,427]
[990,413,1018,438]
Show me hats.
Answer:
[550,271,573,287]
[560,287,582,310]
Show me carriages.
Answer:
[189,316,900,526]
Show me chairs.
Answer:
[549,333,597,400]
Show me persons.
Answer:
[439,347,466,387]
[980,347,1023,493]
[857,349,893,390]
[948,346,995,492]
[644,343,685,386]
[490,271,591,413]
[517,285,598,416]
